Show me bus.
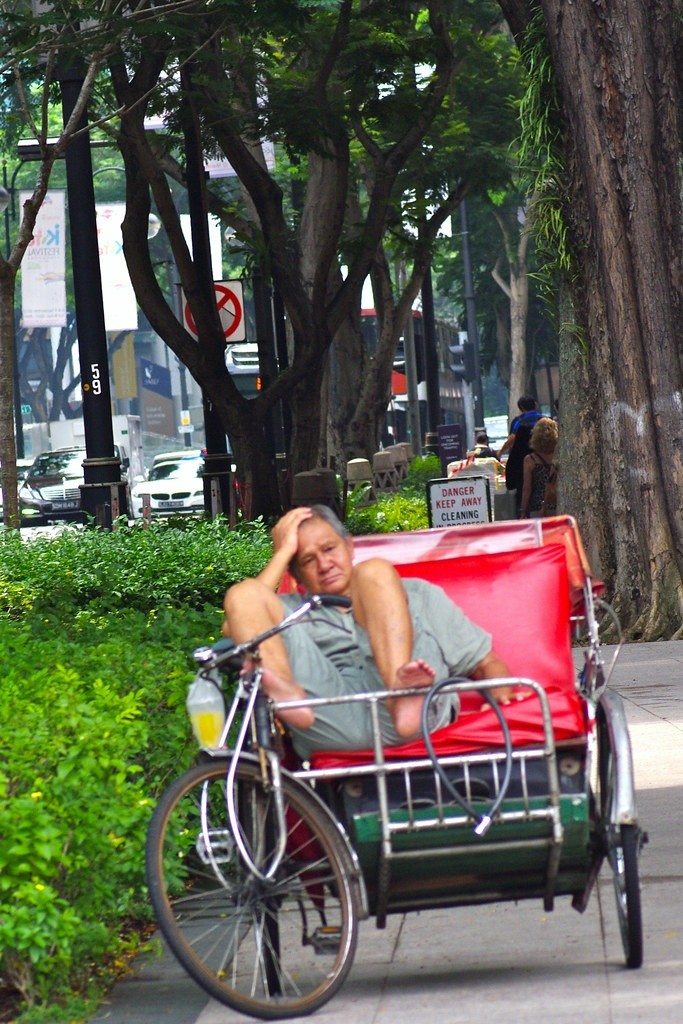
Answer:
[359,304,475,458]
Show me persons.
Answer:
[468,434,500,463]
[497,395,559,522]
[220,504,534,762]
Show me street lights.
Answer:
[163,179,248,454]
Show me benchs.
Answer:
[304,541,584,772]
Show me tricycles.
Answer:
[144,517,654,1021]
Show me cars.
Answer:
[130,450,207,520]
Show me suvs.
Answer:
[17,441,134,526]
